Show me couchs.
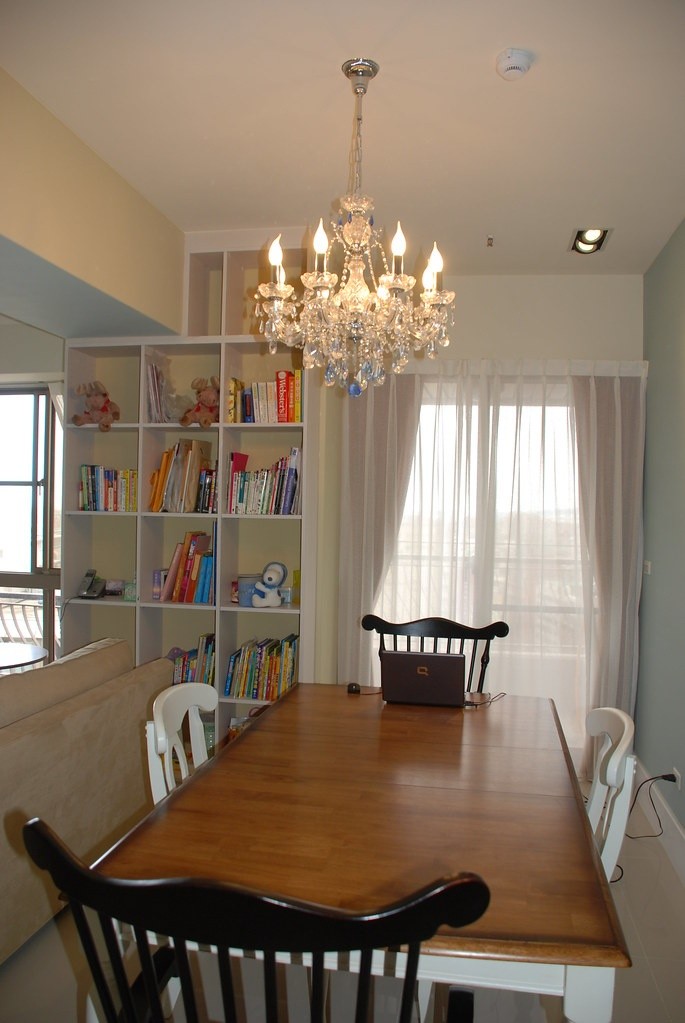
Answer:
[0,640,175,970]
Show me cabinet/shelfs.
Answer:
[50,224,320,758]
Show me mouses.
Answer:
[348,683,360,692]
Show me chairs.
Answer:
[359,613,509,692]
[144,683,218,804]
[22,816,492,1023]
[574,707,639,881]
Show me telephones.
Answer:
[78,568,107,599]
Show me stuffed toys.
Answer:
[252,562,289,607]
[180,376,220,428]
[71,381,120,432]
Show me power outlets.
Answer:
[672,768,681,790]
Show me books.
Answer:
[196,469,218,514]
[172,634,215,685]
[231,447,298,514]
[223,633,300,701]
[159,531,213,603]
[78,464,138,512]
[228,370,301,422]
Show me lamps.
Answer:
[252,55,457,396]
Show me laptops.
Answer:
[381,651,464,705]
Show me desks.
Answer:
[60,683,633,1023]
[0,644,48,670]
